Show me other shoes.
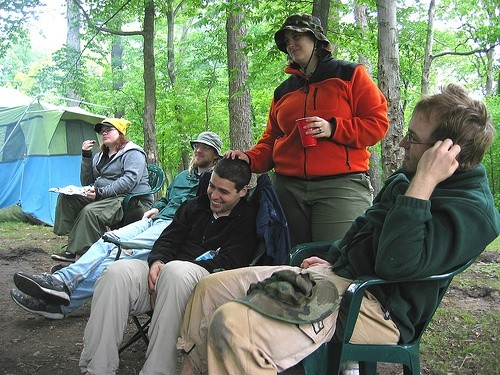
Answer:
[50,264,69,273]
[51,245,75,263]
[341,362,359,375]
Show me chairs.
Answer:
[118,163,166,228]
[284,240,480,375]
[118,184,267,354]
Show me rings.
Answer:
[319,128,323,132]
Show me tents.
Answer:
[0,87,108,227]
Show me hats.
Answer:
[190,132,224,159]
[102,118,132,135]
[94,122,112,134]
[230,269,339,324]
[274,13,330,55]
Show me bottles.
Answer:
[193,247,221,261]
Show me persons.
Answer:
[50,118,155,261]
[224,13,388,241]
[79,158,272,375]
[176,83,500,375]
[11,132,223,319]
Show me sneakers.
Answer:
[14,273,70,306]
[11,289,64,319]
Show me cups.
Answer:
[295,117,317,148]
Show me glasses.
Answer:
[406,132,433,146]
[99,128,116,135]
[193,144,210,150]
